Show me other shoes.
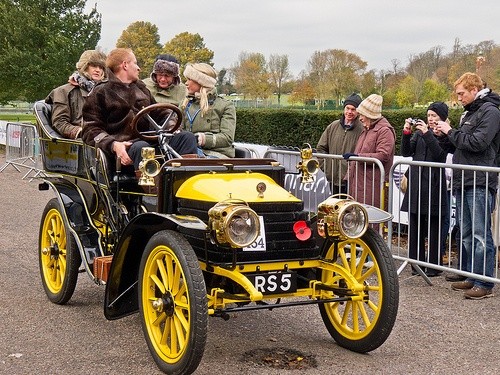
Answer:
[464,284,494,300]
[446,272,468,281]
[425,270,443,276]
[411,267,425,275]
[451,280,476,290]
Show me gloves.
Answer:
[342,153,358,162]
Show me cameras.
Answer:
[410,119,420,127]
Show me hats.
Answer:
[75,50,109,83]
[151,54,182,87]
[183,62,218,88]
[344,93,362,109]
[356,94,383,119]
[427,100,449,122]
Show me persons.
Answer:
[141,54,185,108]
[181,62,236,160]
[402,102,448,276]
[316,93,366,196]
[344,93,395,228]
[46,50,106,139]
[81,48,198,174]
[433,72,500,299]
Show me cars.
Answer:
[33,100,400,375]
